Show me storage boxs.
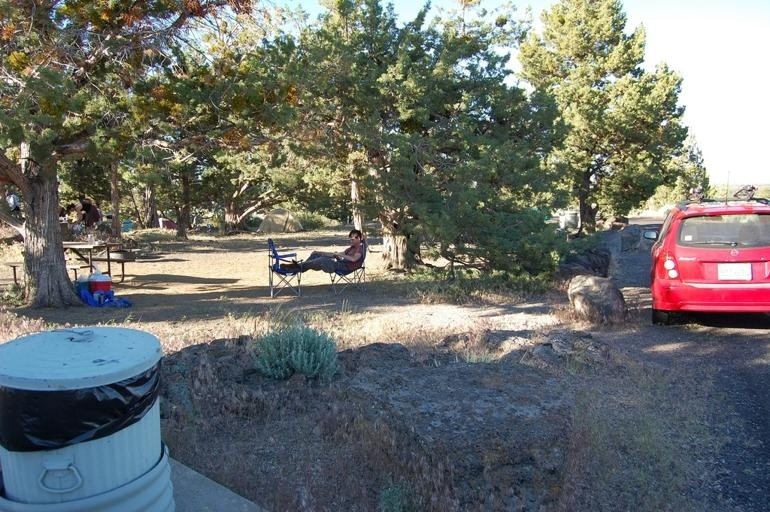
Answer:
[73,278,90,291]
[88,273,112,294]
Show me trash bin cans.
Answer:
[0,326,176,512]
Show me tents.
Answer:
[158,216,176,227]
[123,219,134,231]
[259,209,304,233]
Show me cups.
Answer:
[87,234,95,245]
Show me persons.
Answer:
[279,229,365,274]
[82,198,104,237]
[66,202,102,233]
[160,210,167,217]
[5,191,22,217]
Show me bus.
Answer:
[557,203,580,229]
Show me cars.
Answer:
[643,199,770,325]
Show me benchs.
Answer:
[9,263,90,289]
[74,257,136,286]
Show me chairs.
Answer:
[267,237,304,299]
[325,243,367,297]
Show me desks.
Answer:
[60,239,124,288]
